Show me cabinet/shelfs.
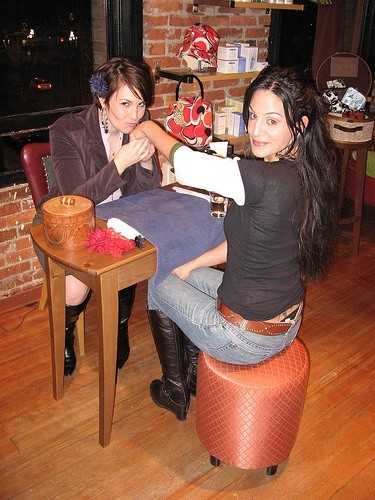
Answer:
[154,0,303,144]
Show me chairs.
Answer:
[22,142,85,358]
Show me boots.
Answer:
[116,283,137,371]
[181,331,200,399]
[63,289,92,378]
[145,304,191,421]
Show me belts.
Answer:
[215,296,304,336]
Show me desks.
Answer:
[325,139,374,255]
[29,182,232,448]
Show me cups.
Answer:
[209,192,228,218]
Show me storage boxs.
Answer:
[214,96,244,138]
[217,41,258,74]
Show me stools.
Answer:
[195,338,310,476]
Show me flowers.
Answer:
[84,227,135,258]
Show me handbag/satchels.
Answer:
[165,75,213,149]
[315,51,375,144]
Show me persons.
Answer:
[31,57,163,380]
[130,64,326,423]
